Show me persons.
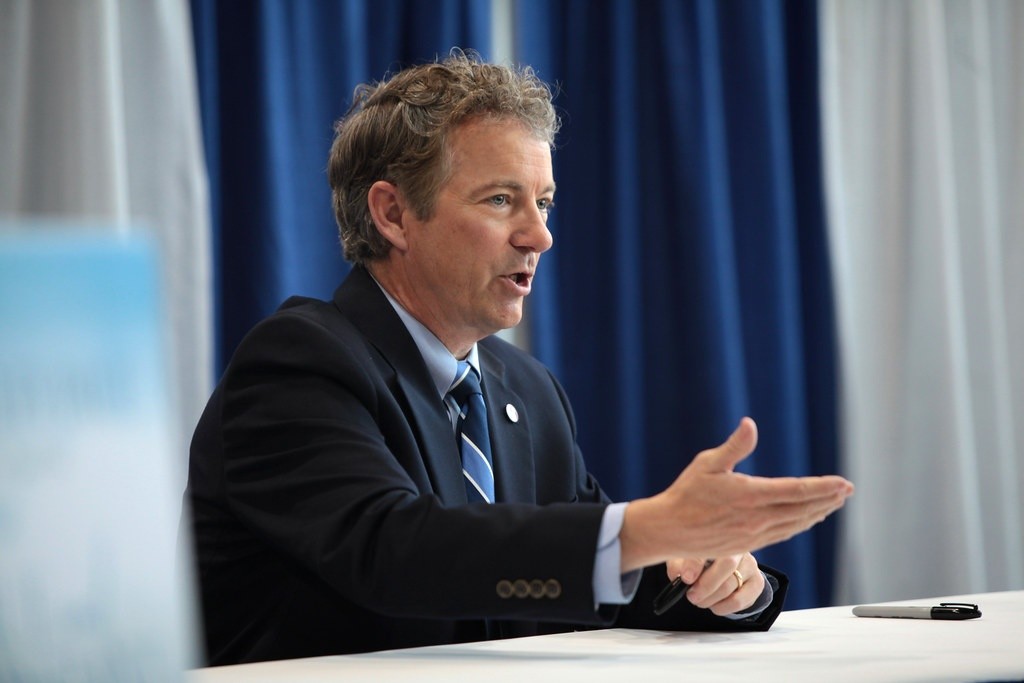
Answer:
[187,41,853,668]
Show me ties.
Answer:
[444,360,494,507]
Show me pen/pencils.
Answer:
[851,603,982,620]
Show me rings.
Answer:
[733,570,743,589]
